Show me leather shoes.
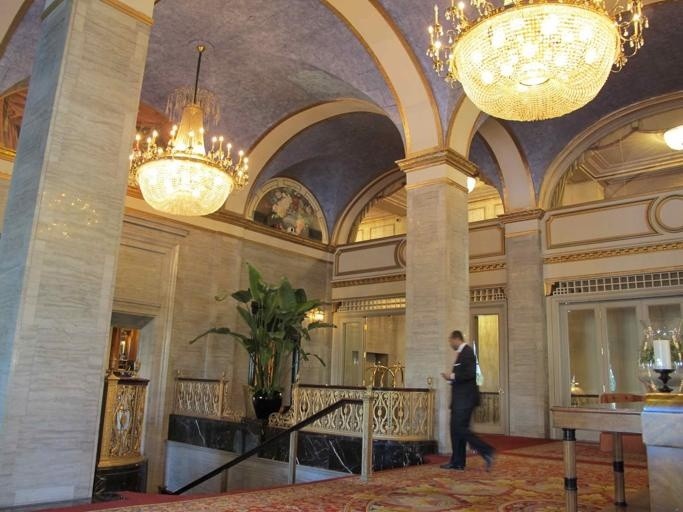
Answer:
[440,465,464,470]
[482,448,496,471]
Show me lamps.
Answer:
[651,339,675,393]
[130,43,250,221]
[424,1,650,126]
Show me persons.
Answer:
[439,330,499,472]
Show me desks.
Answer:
[549,402,683,512]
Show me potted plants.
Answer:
[189,260,340,425]
